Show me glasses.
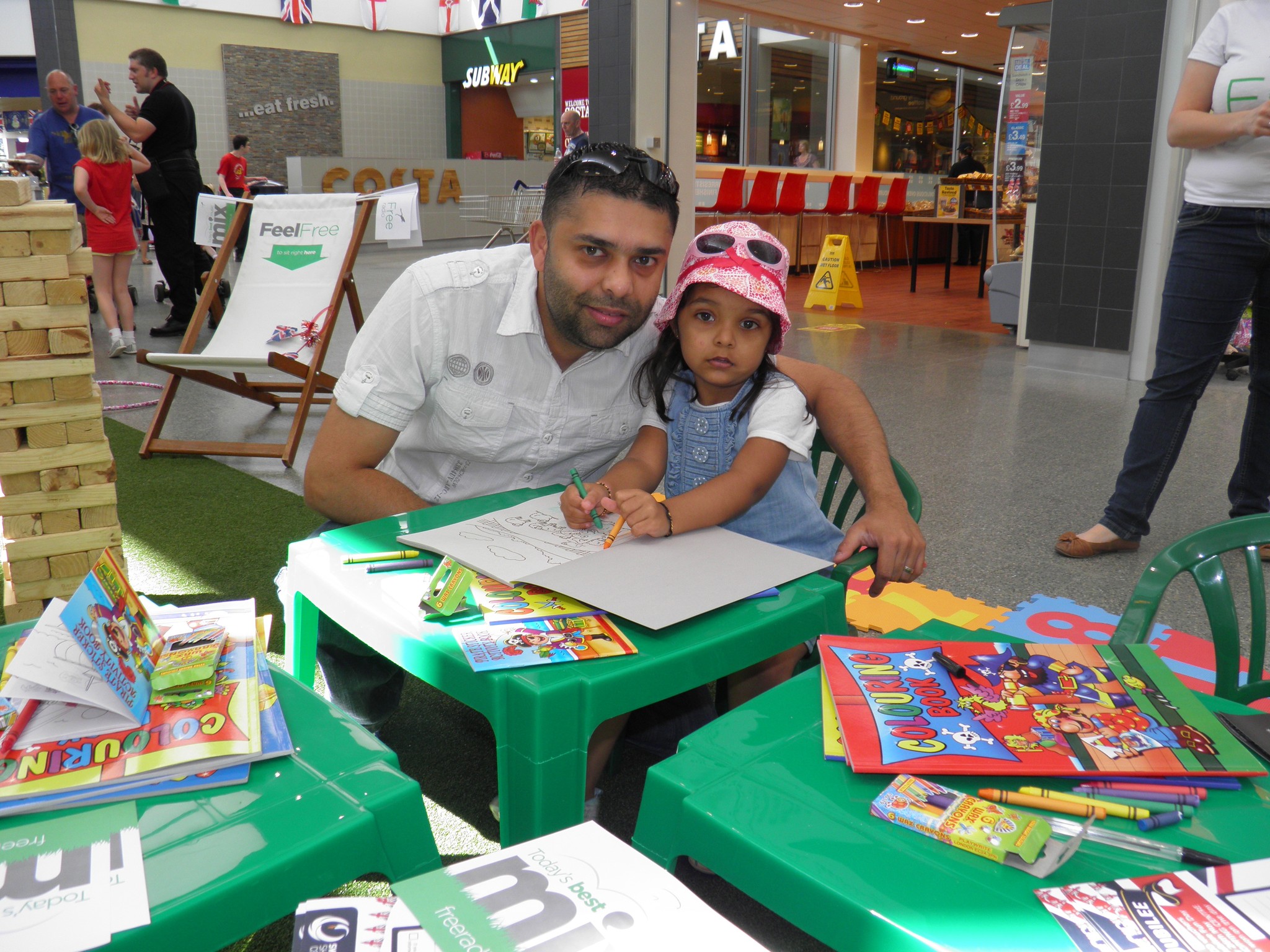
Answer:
[679,233,787,286]
[553,150,679,205]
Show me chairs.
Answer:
[696,168,910,278]
[137,182,425,468]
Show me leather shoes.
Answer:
[206,285,224,329]
[150,317,188,337]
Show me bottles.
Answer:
[555,148,562,166]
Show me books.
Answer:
[815,632,1267,778]
[395,490,834,631]
[462,566,607,625]
[0,547,294,819]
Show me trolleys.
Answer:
[457,180,546,248]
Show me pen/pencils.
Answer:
[1045,814,1233,867]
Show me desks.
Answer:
[902,216,1023,299]
[0,483,1270,952]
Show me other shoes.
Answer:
[108,339,127,357]
[234,249,242,262]
[142,259,153,265]
[971,260,979,264]
[1056,532,1139,558]
[124,342,137,354]
[1235,544,1270,561]
[953,259,967,264]
[117,316,137,332]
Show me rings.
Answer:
[904,566,913,573]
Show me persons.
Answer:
[1054,0,1270,563]
[304,136,927,736]
[200,135,268,260]
[94,47,225,338]
[543,110,589,190]
[487,219,846,874]
[948,142,987,265]
[15,70,153,356]
[793,139,818,168]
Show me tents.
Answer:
[450,615,639,673]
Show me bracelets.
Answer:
[658,502,673,538]
[596,482,612,517]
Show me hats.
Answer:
[653,221,792,357]
[956,142,972,156]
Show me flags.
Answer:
[268,325,299,360]
[0,114,3,133]
[280,0,589,33]
[28,110,39,127]
